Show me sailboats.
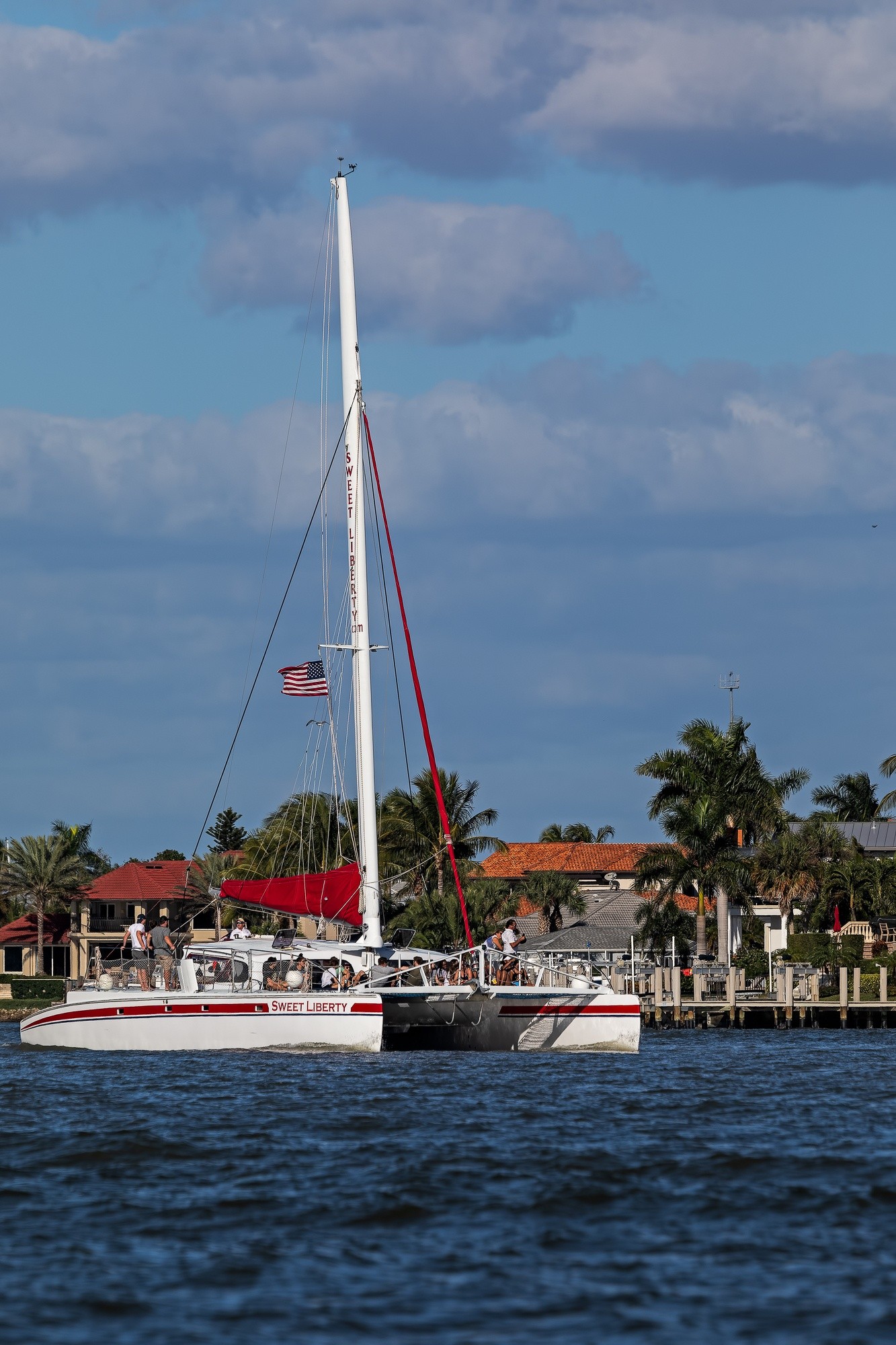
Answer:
[15,173,646,1057]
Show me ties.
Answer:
[344,963,352,966]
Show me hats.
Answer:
[160,916,168,922]
[137,914,146,924]
[236,918,244,922]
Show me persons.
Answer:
[330,956,352,987]
[321,959,345,990]
[466,964,478,979]
[343,962,372,993]
[286,953,312,989]
[453,959,473,985]
[400,956,429,987]
[424,959,444,986]
[229,918,252,940]
[120,914,154,992]
[437,959,449,985]
[501,919,526,960]
[146,916,179,991]
[495,958,515,986]
[263,957,294,991]
[446,959,459,986]
[370,957,400,987]
[482,930,504,985]
[509,960,536,986]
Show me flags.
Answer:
[277,659,329,697]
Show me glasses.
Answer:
[237,922,244,924]
[345,967,351,969]
[498,932,502,935]
